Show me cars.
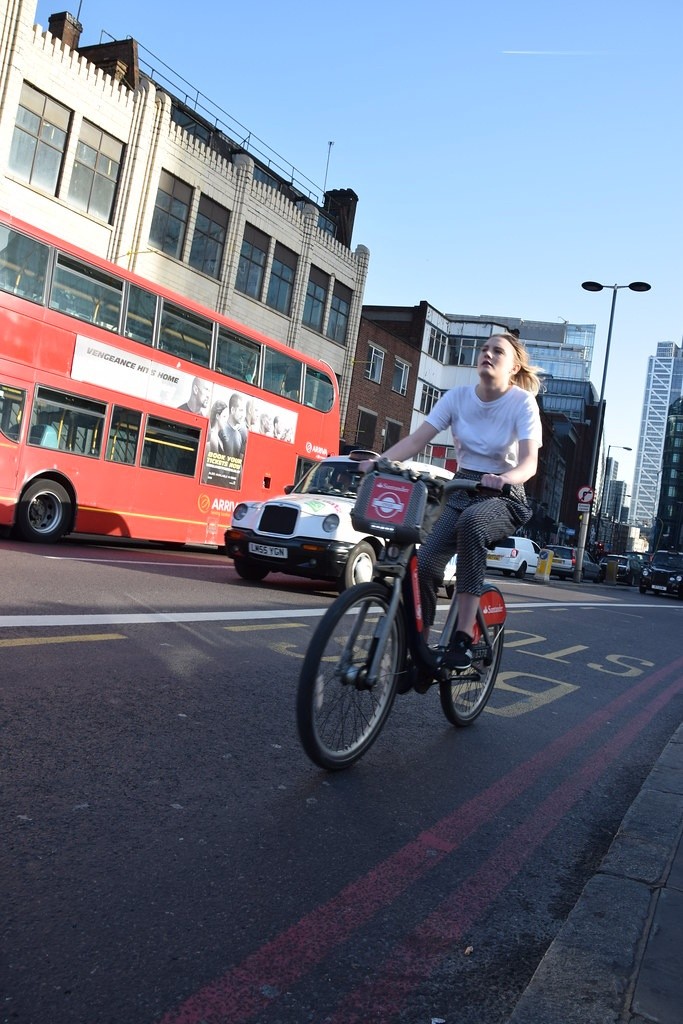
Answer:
[485,537,540,578]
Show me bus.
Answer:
[0,207,340,555]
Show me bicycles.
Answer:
[295,448,525,771]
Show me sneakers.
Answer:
[447,624,478,670]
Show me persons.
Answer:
[353,333,544,673]
[178,376,295,489]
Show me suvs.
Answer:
[544,544,602,583]
[599,551,683,598]
[224,455,457,606]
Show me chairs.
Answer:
[7,423,59,451]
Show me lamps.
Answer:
[381,429,386,437]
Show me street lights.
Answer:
[576,281,651,582]
[595,445,632,540]
[608,494,631,554]
